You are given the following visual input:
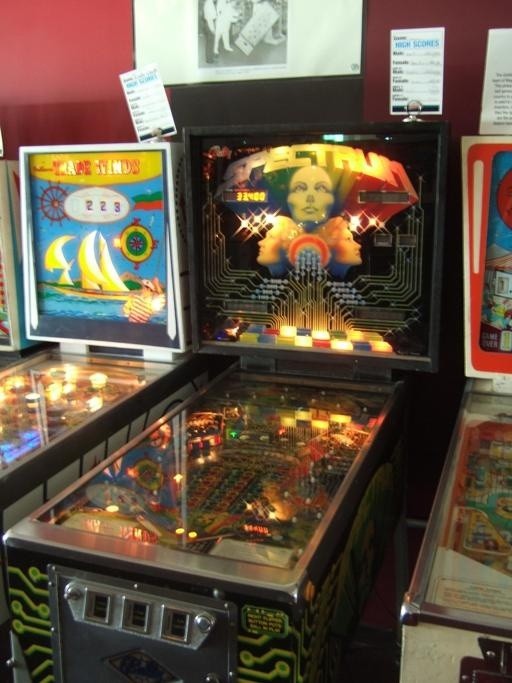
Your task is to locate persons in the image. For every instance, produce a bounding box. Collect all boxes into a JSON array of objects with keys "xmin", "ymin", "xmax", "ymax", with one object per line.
[
  {"xmin": 201, "ymin": 0, "xmax": 239, "ymax": 64},
  {"xmin": 255, "ymin": 164, "xmax": 364, "ymax": 280}
]
